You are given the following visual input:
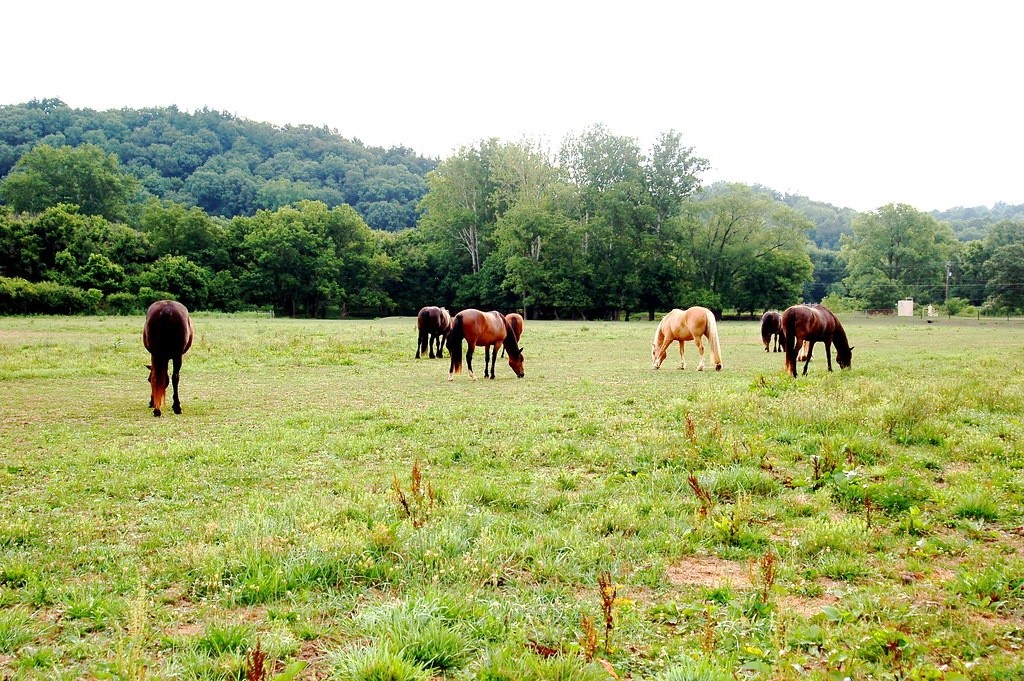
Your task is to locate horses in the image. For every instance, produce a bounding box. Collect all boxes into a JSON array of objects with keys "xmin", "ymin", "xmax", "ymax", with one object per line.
[
  {"xmin": 415, "ymin": 306, "xmax": 525, "ymax": 382},
  {"xmin": 144, "ymin": 300, "xmax": 194, "ymax": 418},
  {"xmin": 650, "ymin": 306, "xmax": 722, "ymax": 372},
  {"xmin": 761, "ymin": 305, "xmax": 855, "ymax": 378}
]
